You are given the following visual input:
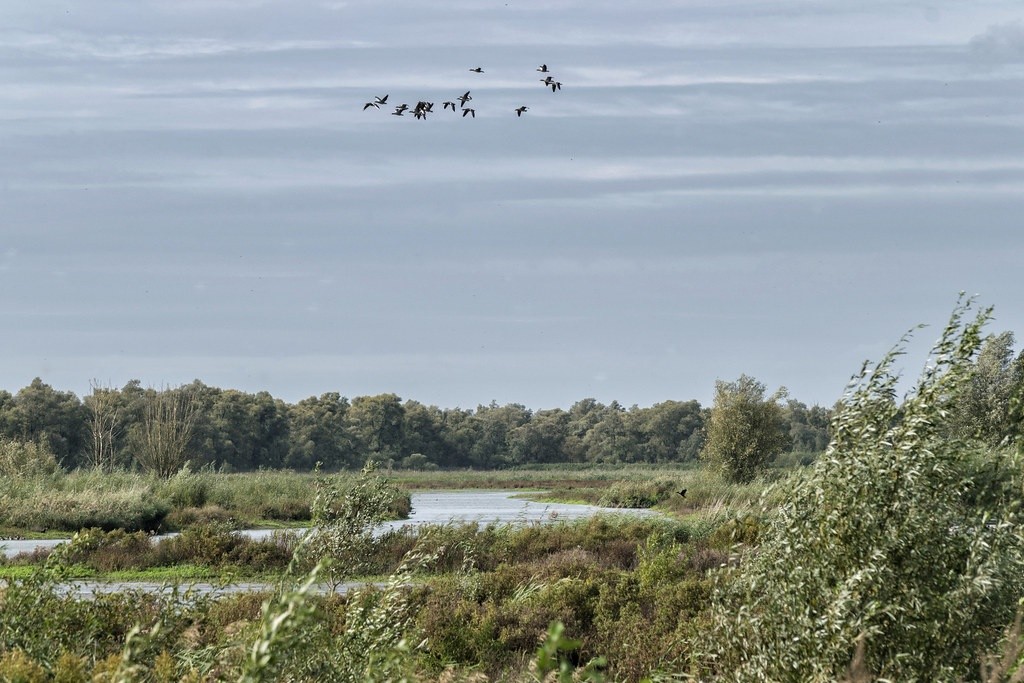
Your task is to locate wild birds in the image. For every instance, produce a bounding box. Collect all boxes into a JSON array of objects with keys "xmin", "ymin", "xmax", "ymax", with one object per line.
[
  {"xmin": 674, "ymin": 488, "xmax": 688, "ymax": 499},
  {"xmin": 362, "ymin": 63, "xmax": 564, "ymax": 121}
]
